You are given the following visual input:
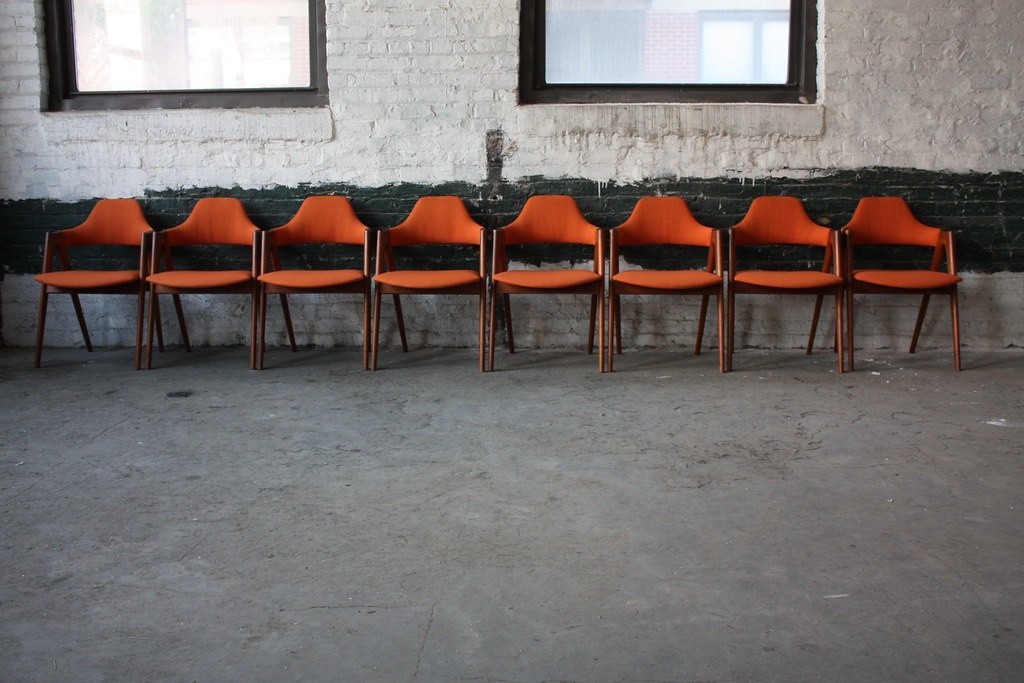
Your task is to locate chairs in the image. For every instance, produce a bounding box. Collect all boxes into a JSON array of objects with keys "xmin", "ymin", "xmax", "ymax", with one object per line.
[
  {"xmin": 33, "ymin": 198, "xmax": 164, "ymax": 371},
  {"xmin": 834, "ymin": 197, "xmax": 963, "ymax": 372},
  {"xmin": 727, "ymin": 197, "xmax": 843, "ymax": 374},
  {"xmin": 607, "ymin": 196, "xmax": 724, "ymax": 374},
  {"xmin": 145, "ymin": 198, "xmax": 266, "ymax": 370},
  {"xmin": 369, "ymin": 196, "xmax": 496, "ymax": 373},
  {"xmin": 489, "ymin": 195, "xmax": 605, "ymax": 374},
  {"xmin": 257, "ymin": 198, "xmax": 371, "ymax": 372}
]
